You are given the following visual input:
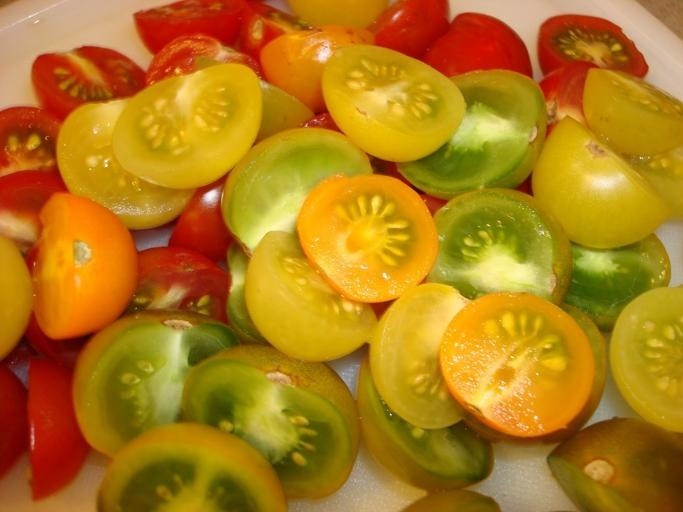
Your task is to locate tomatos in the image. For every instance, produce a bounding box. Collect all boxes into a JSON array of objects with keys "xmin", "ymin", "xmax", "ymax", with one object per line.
[{"xmin": 0, "ymin": 1, "xmax": 683, "ymax": 512}]
